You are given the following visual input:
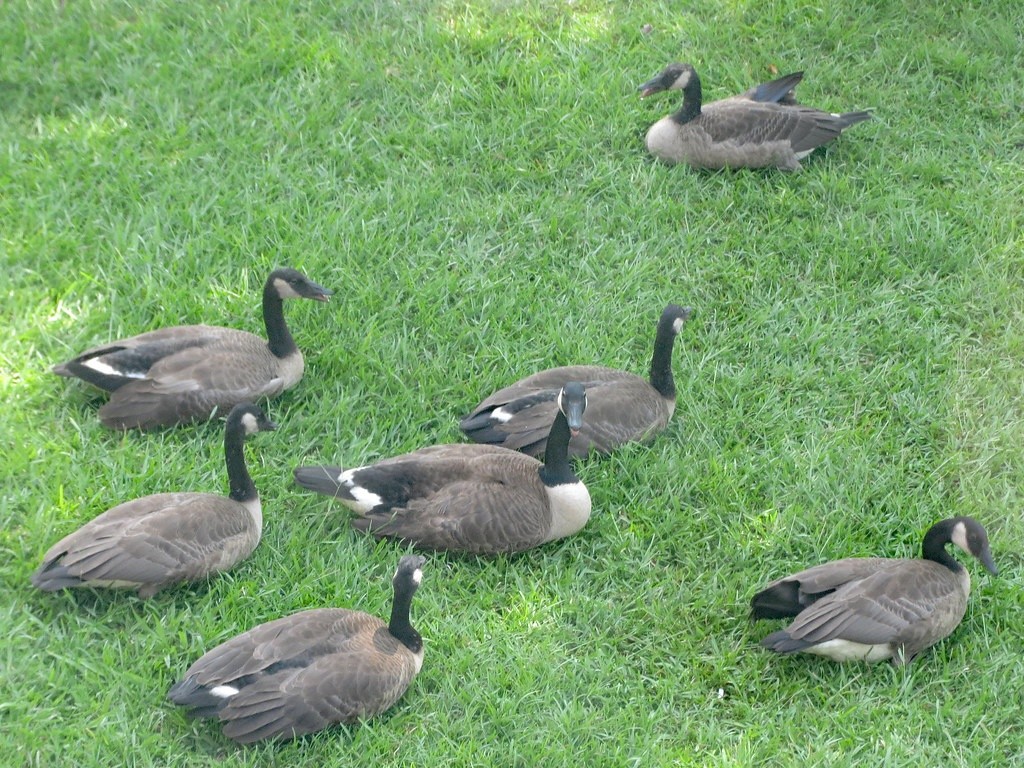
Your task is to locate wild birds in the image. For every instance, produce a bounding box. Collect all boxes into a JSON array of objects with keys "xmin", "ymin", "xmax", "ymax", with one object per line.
[
  {"xmin": 165, "ymin": 554, "xmax": 426, "ymax": 745},
  {"xmin": 51, "ymin": 268, "xmax": 334, "ymax": 432},
  {"xmin": 30, "ymin": 401, "xmax": 280, "ymax": 601},
  {"xmin": 748, "ymin": 517, "xmax": 1000, "ymax": 661},
  {"xmin": 293, "ymin": 382, "xmax": 592, "ymax": 554},
  {"xmin": 460, "ymin": 302, "xmax": 696, "ymax": 463},
  {"xmin": 638, "ymin": 62, "xmax": 876, "ymax": 171}
]
